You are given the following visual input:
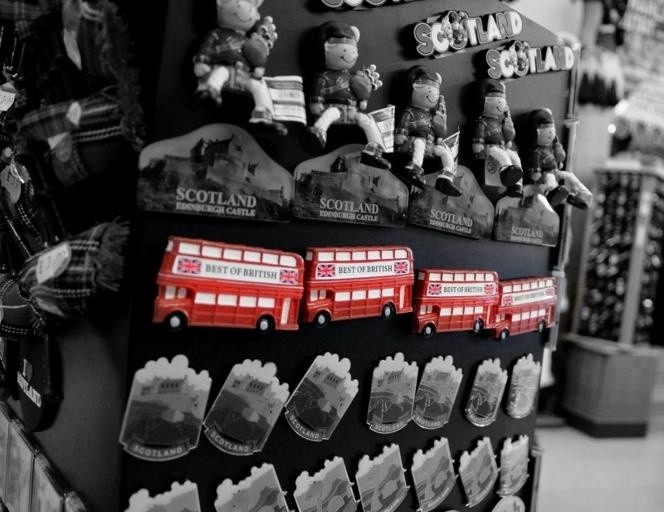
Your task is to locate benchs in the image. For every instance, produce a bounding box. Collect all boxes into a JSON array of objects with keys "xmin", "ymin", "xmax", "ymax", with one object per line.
[
  {"xmin": 484, "ymin": 284, "xmax": 495, "ymax": 293},
  {"xmin": 503, "ymin": 296, "xmax": 513, "ymax": 305},
  {"xmin": 429, "ymin": 283, "xmax": 441, "ymax": 295},
  {"xmin": 177, "ymin": 258, "xmax": 202, "ymax": 275},
  {"xmin": 394, "ymin": 262, "xmax": 408, "ymax": 274},
  {"xmin": 279, "ymin": 269, "xmax": 297, "ymax": 283},
  {"xmin": 318, "ymin": 264, "xmax": 336, "ymax": 277}
]
